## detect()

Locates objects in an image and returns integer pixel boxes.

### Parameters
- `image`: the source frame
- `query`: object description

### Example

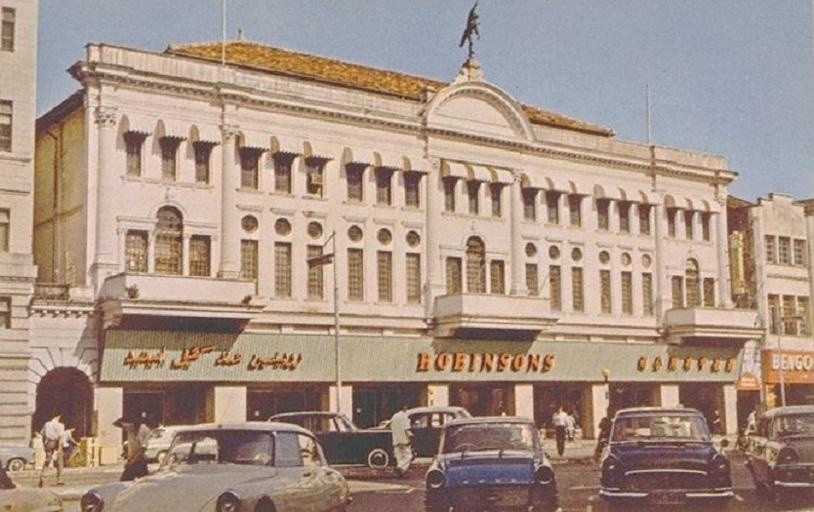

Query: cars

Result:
[120,424,219,463]
[600,407,736,504]
[80,420,352,512]
[0,445,35,474]
[0,468,64,512]
[738,406,814,496]
[267,407,472,469]
[422,414,559,512]
